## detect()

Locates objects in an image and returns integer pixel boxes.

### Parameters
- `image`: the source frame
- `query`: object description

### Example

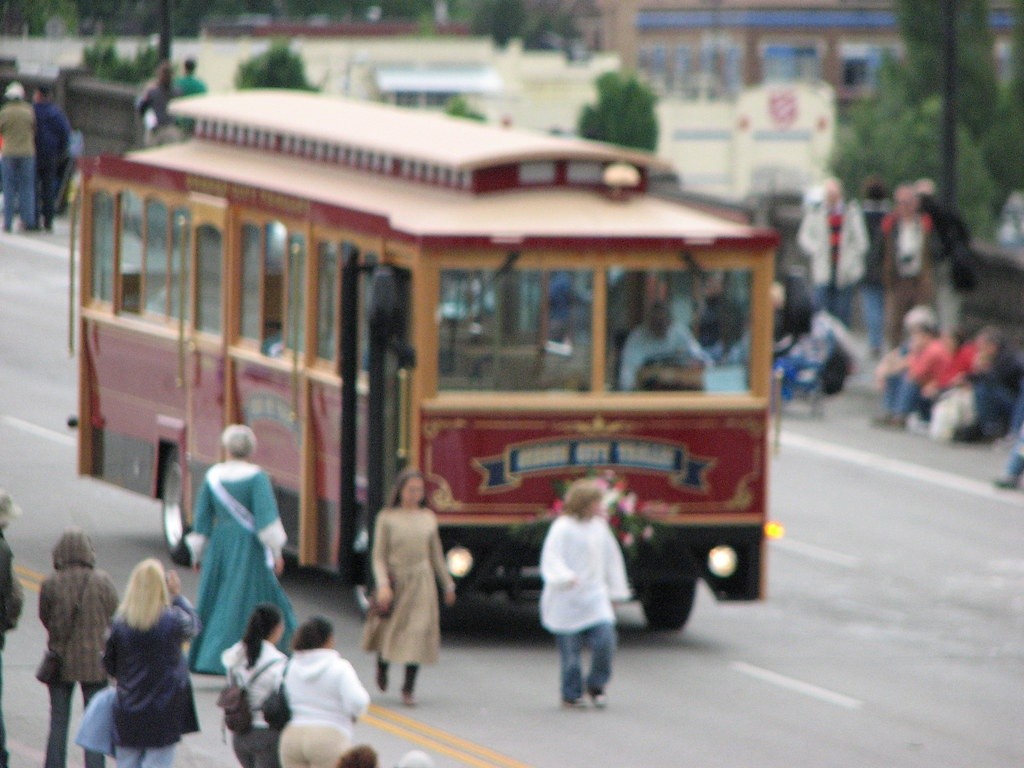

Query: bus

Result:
[67,85,782,634]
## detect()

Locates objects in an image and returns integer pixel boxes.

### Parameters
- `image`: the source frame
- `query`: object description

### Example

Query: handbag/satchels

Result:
[369,577,397,620]
[262,689,290,732]
[36,654,64,686]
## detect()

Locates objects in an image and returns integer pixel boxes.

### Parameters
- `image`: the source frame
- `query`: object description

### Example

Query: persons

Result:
[538,477,634,711]
[0,81,71,231]
[274,616,371,768]
[39,528,121,768]
[534,269,752,390]
[363,470,458,707]
[0,491,23,768]
[220,603,290,768]
[103,558,200,768]
[770,176,1024,488]
[337,744,435,768]
[182,423,299,676]
[139,59,207,147]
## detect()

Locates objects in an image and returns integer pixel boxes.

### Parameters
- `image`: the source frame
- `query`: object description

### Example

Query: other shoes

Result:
[592,694,608,708]
[562,697,587,709]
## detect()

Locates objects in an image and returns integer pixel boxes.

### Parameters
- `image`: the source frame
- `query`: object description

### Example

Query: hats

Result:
[5,83,25,101]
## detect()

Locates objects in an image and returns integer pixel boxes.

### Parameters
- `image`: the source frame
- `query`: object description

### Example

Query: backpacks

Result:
[216,684,253,733]
